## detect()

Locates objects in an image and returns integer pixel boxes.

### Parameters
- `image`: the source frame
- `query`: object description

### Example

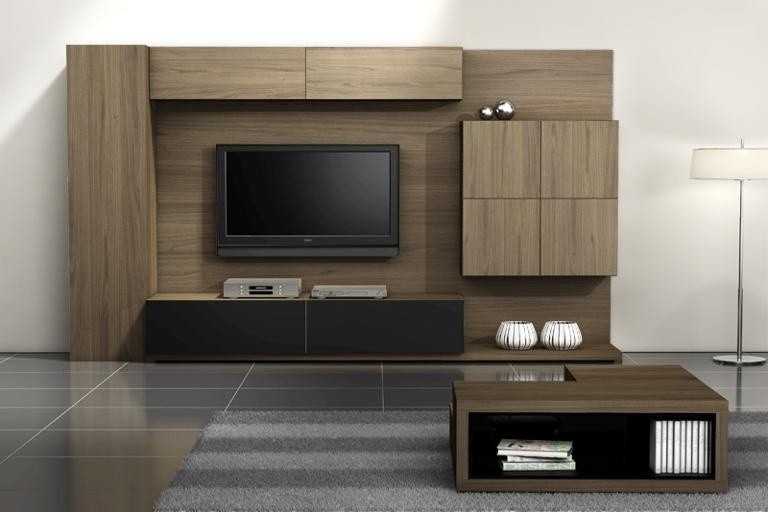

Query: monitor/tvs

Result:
[216,144,399,247]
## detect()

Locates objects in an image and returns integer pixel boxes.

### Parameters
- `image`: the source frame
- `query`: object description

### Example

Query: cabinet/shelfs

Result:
[149,47,462,99]
[145,294,463,355]
[460,120,619,276]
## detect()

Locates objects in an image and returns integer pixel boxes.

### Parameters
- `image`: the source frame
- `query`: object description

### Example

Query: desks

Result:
[450,365,729,494]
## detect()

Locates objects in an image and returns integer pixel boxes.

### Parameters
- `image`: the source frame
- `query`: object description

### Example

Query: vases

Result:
[496,316,538,349]
[541,320,583,350]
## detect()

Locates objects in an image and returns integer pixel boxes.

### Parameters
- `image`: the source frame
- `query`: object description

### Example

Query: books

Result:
[494,436,581,472]
[647,418,714,475]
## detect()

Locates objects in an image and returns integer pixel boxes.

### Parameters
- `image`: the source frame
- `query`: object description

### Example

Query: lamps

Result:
[689,140,768,365]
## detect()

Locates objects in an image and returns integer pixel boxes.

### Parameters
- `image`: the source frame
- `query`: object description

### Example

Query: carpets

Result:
[150,411,768,511]
